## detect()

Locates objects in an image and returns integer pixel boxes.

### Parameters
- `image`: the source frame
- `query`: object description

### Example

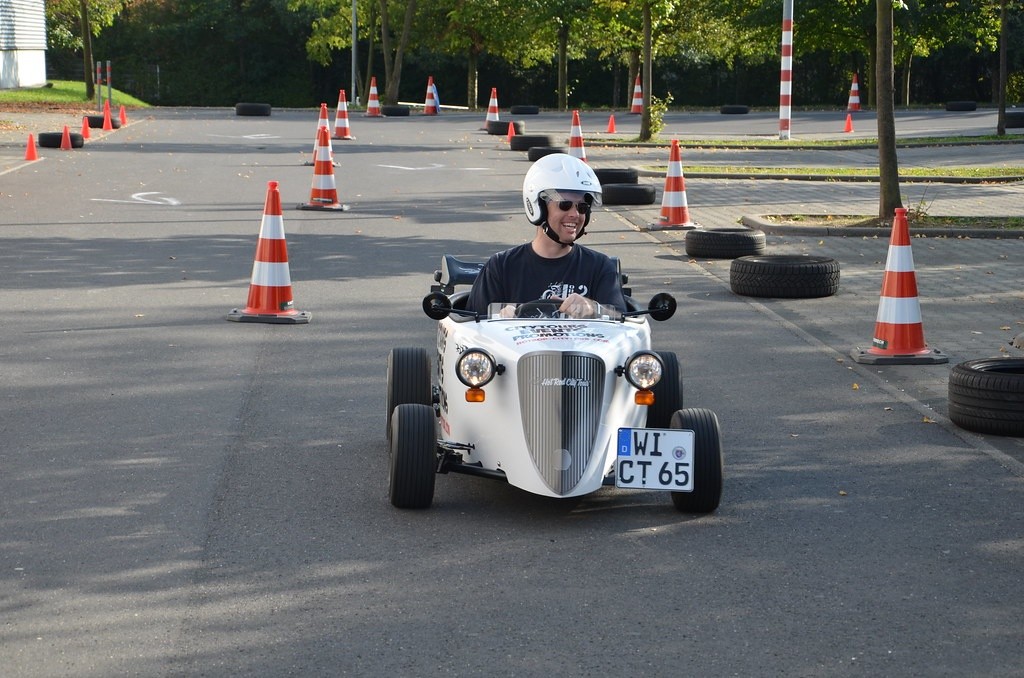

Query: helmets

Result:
[523,153,603,226]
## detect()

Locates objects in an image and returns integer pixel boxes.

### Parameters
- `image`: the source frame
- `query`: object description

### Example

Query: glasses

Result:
[553,199,590,214]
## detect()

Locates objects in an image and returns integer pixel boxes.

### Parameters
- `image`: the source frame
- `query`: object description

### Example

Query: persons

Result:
[466,152,628,322]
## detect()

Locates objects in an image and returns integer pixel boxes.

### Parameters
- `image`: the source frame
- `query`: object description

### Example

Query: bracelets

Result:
[594,301,601,320]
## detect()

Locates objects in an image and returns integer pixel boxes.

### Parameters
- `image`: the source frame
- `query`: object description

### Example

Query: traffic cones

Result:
[626,76,643,115]
[301,103,341,166]
[605,115,619,134]
[478,88,500,130]
[100,99,113,131]
[80,117,91,139]
[567,110,587,163]
[330,90,357,139]
[226,179,313,324]
[417,76,442,116]
[60,125,73,150]
[648,139,703,230]
[118,105,127,125]
[362,78,385,117]
[296,125,349,211]
[842,114,855,132]
[843,73,866,113]
[504,120,515,142]
[848,206,949,365]
[25,134,40,160]
